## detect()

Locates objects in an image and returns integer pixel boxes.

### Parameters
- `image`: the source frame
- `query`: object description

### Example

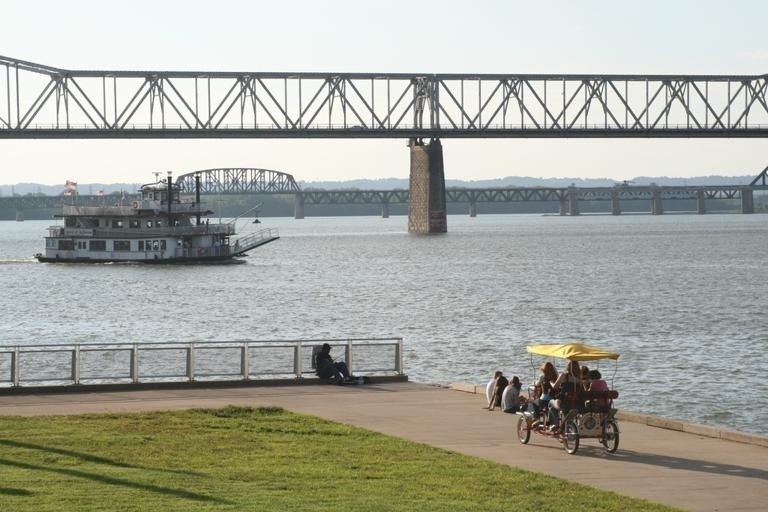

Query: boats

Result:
[34,167,283,264]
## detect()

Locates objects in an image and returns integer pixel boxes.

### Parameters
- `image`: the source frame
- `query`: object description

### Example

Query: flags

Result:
[63,180,77,198]
[96,190,104,197]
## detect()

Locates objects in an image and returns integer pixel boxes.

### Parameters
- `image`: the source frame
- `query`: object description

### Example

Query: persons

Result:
[482,357,612,434]
[313,343,355,386]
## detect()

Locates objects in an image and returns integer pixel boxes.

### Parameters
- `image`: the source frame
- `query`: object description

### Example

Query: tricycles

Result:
[513,343,621,456]
